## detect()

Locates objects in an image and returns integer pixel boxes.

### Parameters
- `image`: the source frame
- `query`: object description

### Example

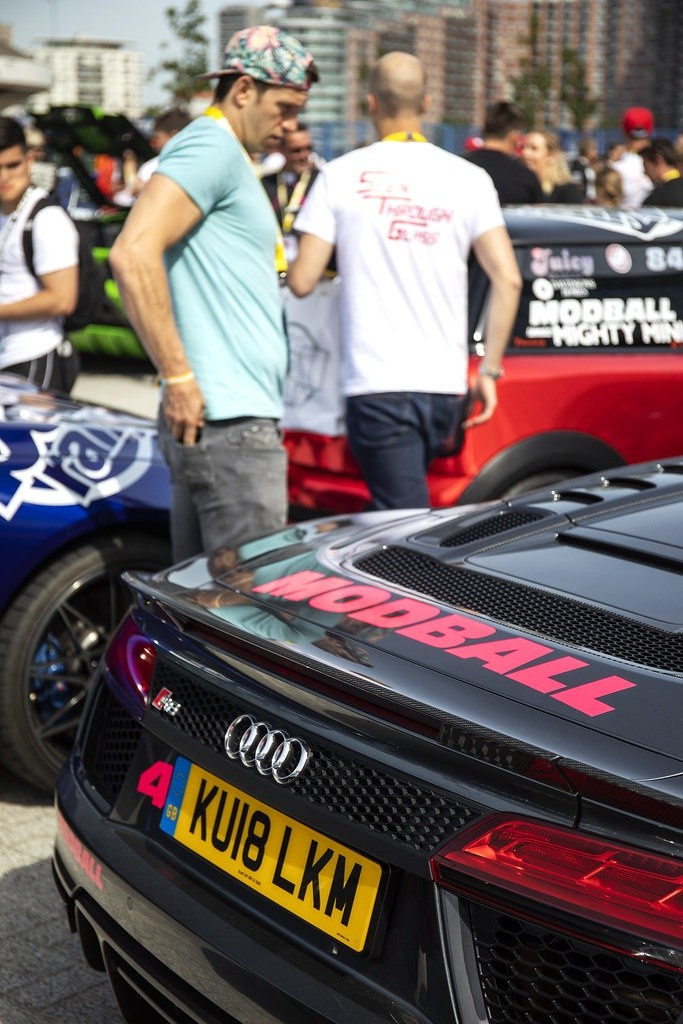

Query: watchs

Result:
[477,364,504,381]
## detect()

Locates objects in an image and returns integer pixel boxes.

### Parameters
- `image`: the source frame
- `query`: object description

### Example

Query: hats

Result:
[621,106,655,138]
[192,26,319,91]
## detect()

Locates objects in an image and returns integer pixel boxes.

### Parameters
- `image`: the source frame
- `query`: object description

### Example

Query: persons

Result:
[257,121,339,287]
[288,50,524,511]
[0,104,197,400]
[462,100,681,352]
[108,25,291,564]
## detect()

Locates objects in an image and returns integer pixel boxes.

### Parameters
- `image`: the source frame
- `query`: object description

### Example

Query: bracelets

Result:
[154,371,195,388]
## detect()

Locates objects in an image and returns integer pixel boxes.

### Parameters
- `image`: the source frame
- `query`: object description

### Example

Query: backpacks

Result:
[23,196,100,330]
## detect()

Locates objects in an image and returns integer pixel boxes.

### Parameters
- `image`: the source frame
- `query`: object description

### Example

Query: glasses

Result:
[289,145,314,153]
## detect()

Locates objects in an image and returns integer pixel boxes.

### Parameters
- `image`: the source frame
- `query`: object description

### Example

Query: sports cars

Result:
[52,455,682,1017]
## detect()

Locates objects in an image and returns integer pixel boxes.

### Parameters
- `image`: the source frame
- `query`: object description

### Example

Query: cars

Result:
[0,373,173,799]
[280,203,683,539]
